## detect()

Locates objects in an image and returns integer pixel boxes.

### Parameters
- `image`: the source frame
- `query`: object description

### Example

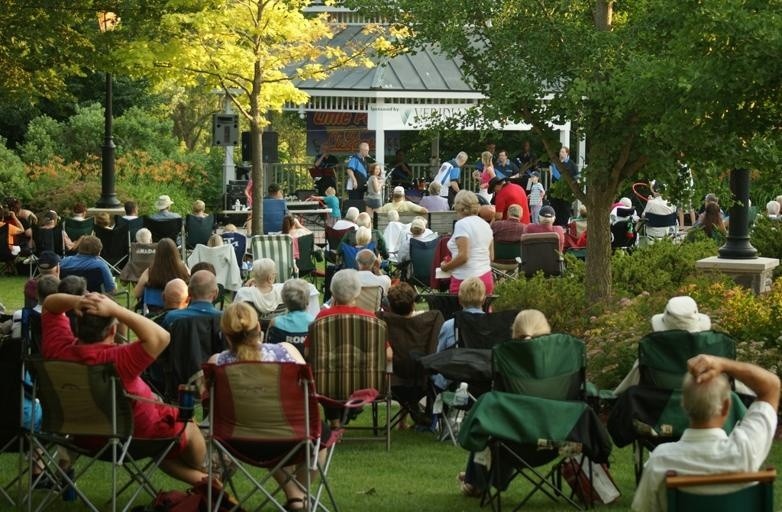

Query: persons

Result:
[512,308,551,340]
[551,145,579,184]
[422,277,487,430]
[366,163,384,209]
[514,139,541,169]
[314,143,339,169]
[434,152,590,295]
[766,196,782,218]
[2,174,438,510]
[494,149,519,176]
[607,178,729,248]
[344,142,370,200]
[474,142,497,172]
[612,296,712,395]
[631,354,782,512]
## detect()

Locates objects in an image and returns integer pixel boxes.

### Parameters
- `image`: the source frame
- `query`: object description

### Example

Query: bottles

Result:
[449,381,470,422]
[242,262,249,276]
[62,467,78,500]
[177,231,182,246]
[236,199,240,211]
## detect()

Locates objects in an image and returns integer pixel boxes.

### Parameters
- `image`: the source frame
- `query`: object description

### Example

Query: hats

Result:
[155,195,173,211]
[488,176,506,194]
[394,186,405,194]
[36,251,62,270]
[539,206,555,217]
[530,171,540,177]
[651,296,712,334]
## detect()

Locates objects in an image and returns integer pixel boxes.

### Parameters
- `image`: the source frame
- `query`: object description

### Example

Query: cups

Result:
[177,383,196,422]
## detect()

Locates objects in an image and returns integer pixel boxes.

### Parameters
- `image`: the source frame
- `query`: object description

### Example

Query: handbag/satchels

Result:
[552,452,622,506]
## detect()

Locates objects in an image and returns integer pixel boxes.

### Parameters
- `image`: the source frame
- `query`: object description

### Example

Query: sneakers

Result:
[458,471,466,481]
[461,480,474,497]
[211,449,241,487]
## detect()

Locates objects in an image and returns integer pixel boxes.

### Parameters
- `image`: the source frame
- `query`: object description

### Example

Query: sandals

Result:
[287,497,308,512]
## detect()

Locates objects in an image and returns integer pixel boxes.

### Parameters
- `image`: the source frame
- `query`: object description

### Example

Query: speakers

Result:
[242,130,277,164]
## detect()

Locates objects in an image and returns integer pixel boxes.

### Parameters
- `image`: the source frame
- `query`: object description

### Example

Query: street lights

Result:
[86,5,128,207]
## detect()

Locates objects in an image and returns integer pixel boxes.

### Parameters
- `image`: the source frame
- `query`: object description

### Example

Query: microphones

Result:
[367,154,376,161]
[516,158,522,164]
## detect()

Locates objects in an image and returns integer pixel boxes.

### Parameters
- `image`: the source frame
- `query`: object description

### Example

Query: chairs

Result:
[1,199,777,509]
